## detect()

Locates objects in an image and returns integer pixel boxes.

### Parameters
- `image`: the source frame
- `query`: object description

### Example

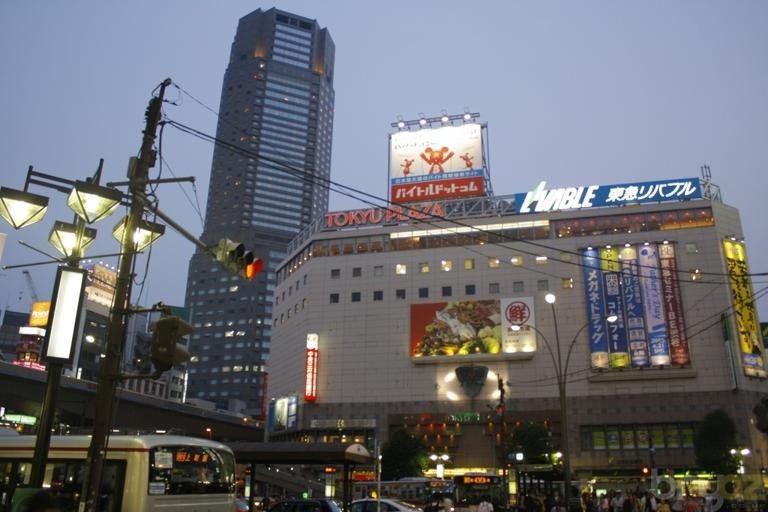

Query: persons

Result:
[429,486,714,512]
[260,496,270,512]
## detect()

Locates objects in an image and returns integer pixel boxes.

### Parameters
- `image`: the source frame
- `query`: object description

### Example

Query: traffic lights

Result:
[214,235,266,283]
[303,492,308,500]
[324,467,337,474]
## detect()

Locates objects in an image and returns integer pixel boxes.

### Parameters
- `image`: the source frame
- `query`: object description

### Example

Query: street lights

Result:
[0,157,166,512]
[510,292,617,512]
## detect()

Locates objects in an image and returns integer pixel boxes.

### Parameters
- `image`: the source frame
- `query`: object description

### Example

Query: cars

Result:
[266,497,343,512]
[344,495,423,512]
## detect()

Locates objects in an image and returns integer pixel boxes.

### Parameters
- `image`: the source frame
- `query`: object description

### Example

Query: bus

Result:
[451,471,501,512]
[1,423,239,508]
[347,475,458,505]
